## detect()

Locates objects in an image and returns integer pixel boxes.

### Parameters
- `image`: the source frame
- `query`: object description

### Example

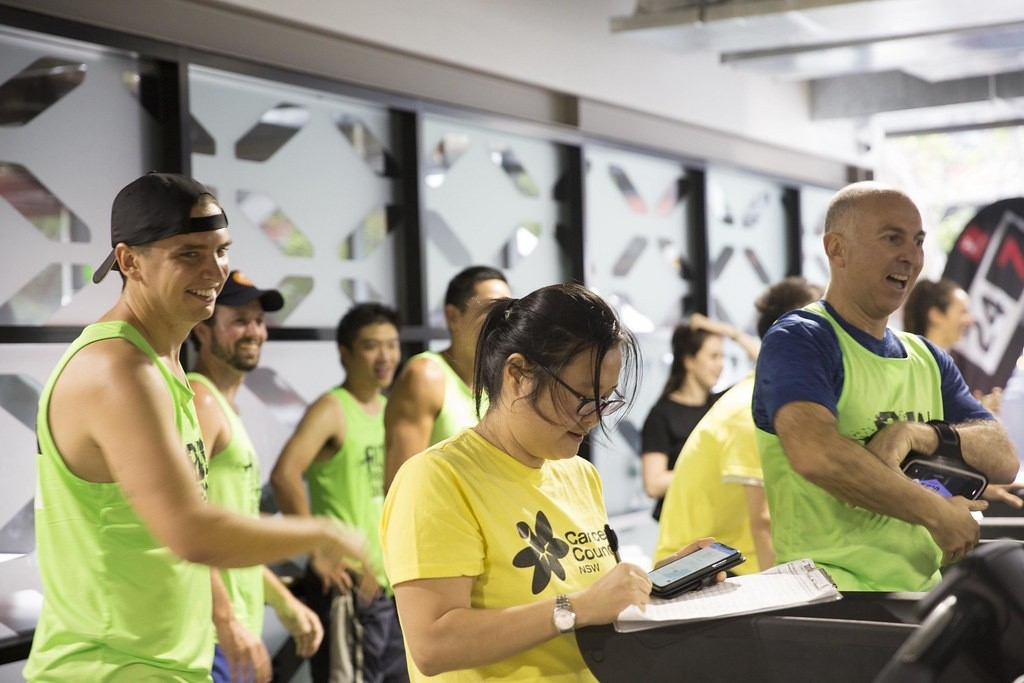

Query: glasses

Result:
[534,358,628,417]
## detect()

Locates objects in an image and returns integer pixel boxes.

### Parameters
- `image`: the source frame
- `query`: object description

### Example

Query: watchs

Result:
[927,421,958,458]
[552,594,575,634]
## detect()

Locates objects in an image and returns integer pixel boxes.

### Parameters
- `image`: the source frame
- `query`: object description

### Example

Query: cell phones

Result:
[647,541,741,593]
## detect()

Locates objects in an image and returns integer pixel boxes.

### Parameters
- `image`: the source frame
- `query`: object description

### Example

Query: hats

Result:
[92,170,230,283]
[215,271,286,311]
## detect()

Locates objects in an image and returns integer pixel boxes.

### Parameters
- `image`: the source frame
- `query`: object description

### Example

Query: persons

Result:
[384,266,514,498]
[379,283,726,683]
[755,280,826,338]
[904,279,972,350]
[654,374,776,579]
[184,268,323,683]
[20,168,368,683]
[750,181,1021,593]
[269,302,399,683]
[640,314,763,523]
